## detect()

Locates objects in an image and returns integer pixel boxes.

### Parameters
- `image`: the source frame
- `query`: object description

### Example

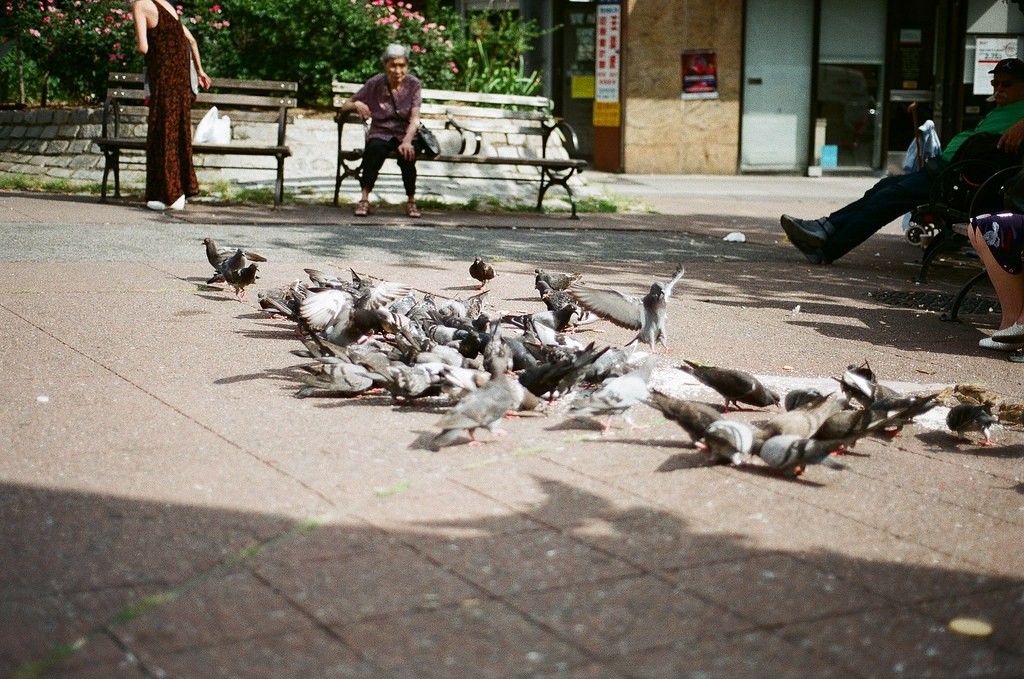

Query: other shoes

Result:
[147,194,185,210]
[978,321,1024,352]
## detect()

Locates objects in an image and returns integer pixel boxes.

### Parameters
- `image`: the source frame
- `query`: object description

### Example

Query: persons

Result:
[341,42,425,219]
[966,204,1024,353]
[778,58,1024,266]
[132,0,213,212]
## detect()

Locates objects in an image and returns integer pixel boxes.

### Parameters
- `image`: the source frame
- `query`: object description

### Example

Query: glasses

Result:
[991,80,1022,88]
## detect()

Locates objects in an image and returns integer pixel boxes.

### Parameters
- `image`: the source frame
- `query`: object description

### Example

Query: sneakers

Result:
[781,214,829,265]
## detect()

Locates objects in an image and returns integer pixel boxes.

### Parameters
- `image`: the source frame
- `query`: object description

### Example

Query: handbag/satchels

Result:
[194,106,231,147]
[190,53,198,99]
[415,125,441,156]
[143,60,198,106]
[999,169,1024,214]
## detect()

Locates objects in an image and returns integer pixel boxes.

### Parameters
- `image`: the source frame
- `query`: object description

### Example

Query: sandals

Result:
[355,200,370,216]
[407,201,421,218]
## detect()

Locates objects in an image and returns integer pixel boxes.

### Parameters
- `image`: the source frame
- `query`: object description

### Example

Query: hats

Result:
[988,58,1024,80]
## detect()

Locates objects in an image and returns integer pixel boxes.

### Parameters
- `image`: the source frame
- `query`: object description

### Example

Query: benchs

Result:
[332,82,588,221]
[919,158,1024,322]
[89,71,298,212]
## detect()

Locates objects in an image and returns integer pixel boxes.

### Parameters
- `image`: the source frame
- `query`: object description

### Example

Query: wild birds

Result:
[199,235,998,478]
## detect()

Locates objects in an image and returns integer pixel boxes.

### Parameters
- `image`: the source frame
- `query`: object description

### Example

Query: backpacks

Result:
[941,132,1024,211]
